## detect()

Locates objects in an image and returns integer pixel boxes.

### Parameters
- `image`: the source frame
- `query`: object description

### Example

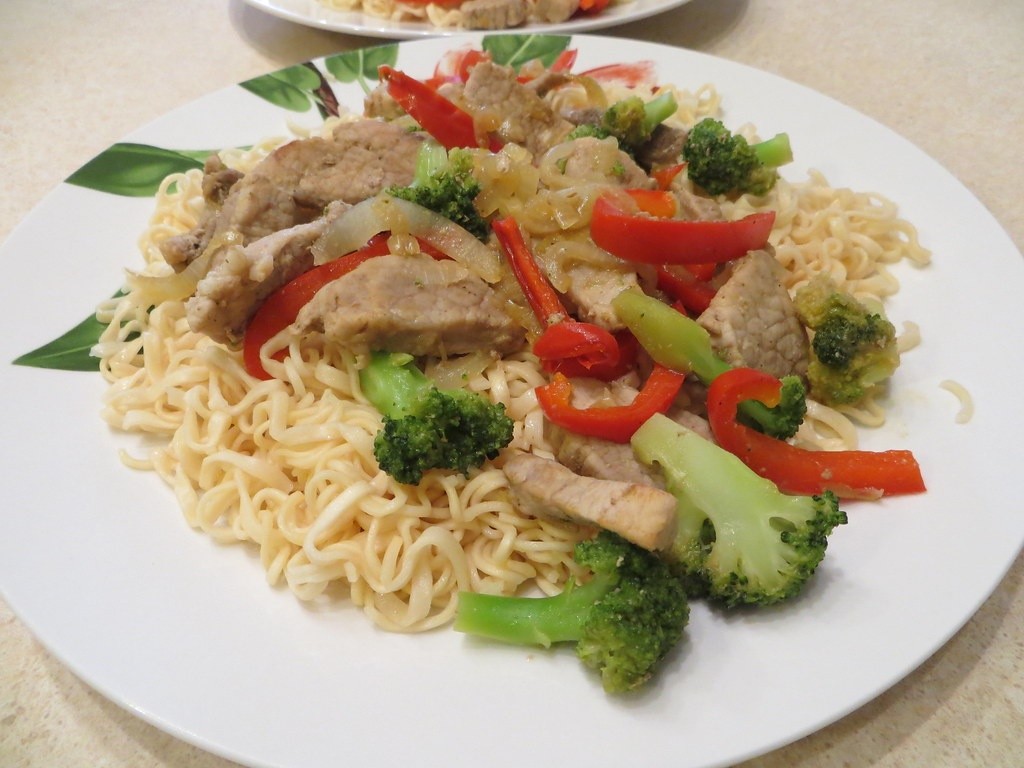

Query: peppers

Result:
[236,53,927,499]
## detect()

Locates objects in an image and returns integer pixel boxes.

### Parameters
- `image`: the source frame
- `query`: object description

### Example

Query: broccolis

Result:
[356,91,904,697]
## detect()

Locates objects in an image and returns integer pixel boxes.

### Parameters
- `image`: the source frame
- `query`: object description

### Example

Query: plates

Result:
[1,35,1024,768]
[243,0,687,39]
